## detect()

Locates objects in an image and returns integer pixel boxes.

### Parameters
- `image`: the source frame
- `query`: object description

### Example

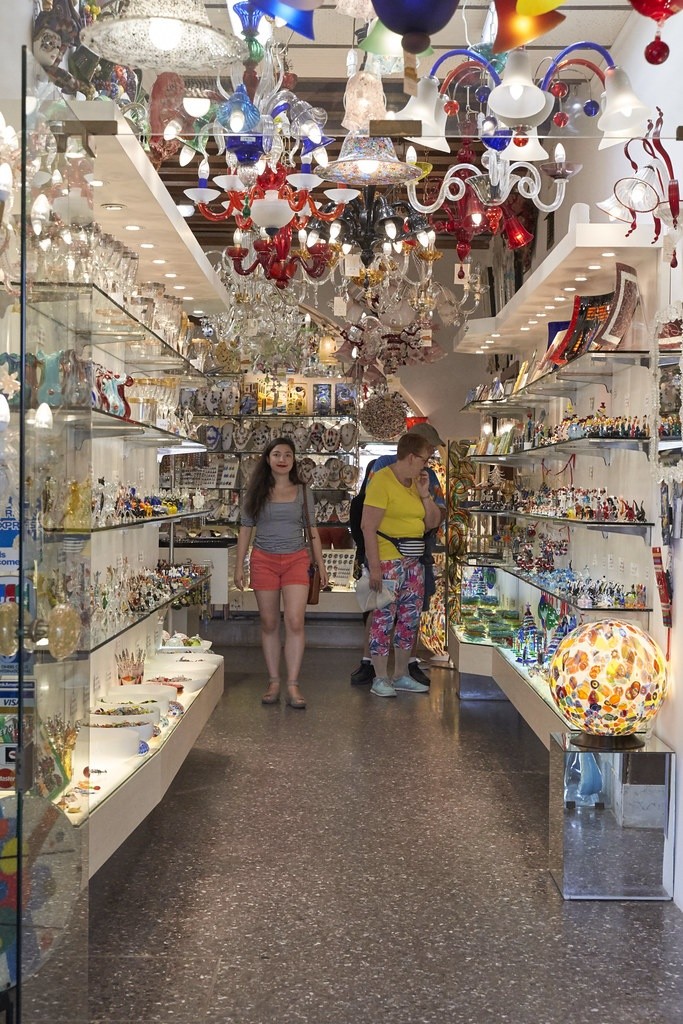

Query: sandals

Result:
[286,680,306,708]
[262,677,281,704]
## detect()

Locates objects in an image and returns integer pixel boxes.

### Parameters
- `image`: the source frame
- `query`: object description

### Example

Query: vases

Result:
[2,353,135,418]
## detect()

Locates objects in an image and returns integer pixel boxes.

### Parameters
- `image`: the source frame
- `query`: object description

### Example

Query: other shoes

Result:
[350,660,376,684]
[370,676,397,696]
[391,674,429,692]
[408,661,431,686]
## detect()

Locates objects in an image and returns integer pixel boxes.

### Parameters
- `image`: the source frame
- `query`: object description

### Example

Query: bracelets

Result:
[420,492,432,500]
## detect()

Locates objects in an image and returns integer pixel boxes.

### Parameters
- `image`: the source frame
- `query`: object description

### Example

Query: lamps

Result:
[0,0,683,370]
[550,617,671,749]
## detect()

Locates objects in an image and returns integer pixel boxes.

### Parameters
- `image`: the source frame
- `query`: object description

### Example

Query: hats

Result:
[409,423,446,448]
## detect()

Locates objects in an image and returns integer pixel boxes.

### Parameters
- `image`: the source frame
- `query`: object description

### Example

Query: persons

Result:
[350,434,436,697]
[318,456,351,551]
[349,422,446,686]
[233,438,329,708]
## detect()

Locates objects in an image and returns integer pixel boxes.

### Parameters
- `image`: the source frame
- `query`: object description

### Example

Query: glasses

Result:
[413,454,428,465]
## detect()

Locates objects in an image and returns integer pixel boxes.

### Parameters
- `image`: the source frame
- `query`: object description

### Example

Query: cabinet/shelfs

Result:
[1,277,223,992]
[158,376,363,619]
[447,201,675,747]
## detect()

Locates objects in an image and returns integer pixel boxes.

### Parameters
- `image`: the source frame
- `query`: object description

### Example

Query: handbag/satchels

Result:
[306,563,321,605]
[378,531,426,556]
